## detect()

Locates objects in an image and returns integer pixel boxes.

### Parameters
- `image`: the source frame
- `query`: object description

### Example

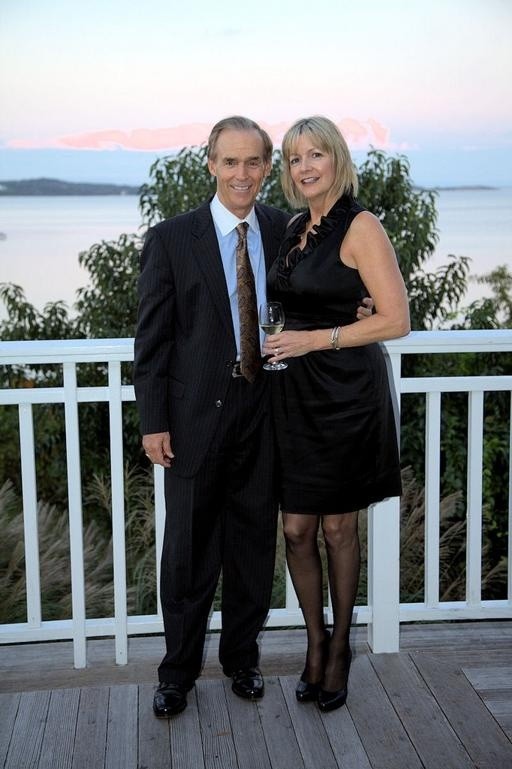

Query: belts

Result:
[231,353,273,378]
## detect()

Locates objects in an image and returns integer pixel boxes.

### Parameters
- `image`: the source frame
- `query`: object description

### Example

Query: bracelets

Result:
[328,326,343,351]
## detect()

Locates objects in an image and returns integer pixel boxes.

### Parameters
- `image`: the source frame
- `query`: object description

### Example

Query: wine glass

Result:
[257,302,289,372]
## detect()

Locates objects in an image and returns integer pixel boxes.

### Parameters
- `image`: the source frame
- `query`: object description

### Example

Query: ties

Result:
[235,222,264,388]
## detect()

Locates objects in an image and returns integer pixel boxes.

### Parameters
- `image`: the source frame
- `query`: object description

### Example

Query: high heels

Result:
[318,646,352,711]
[295,628,332,701]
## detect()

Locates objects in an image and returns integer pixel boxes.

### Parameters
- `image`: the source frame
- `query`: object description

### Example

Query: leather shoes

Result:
[152,679,194,718]
[221,666,266,701]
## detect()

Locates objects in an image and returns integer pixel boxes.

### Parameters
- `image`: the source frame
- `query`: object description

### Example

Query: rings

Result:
[273,347,279,354]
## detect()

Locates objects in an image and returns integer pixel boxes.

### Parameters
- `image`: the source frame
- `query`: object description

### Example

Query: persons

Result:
[261,116,411,711]
[135,116,373,721]
